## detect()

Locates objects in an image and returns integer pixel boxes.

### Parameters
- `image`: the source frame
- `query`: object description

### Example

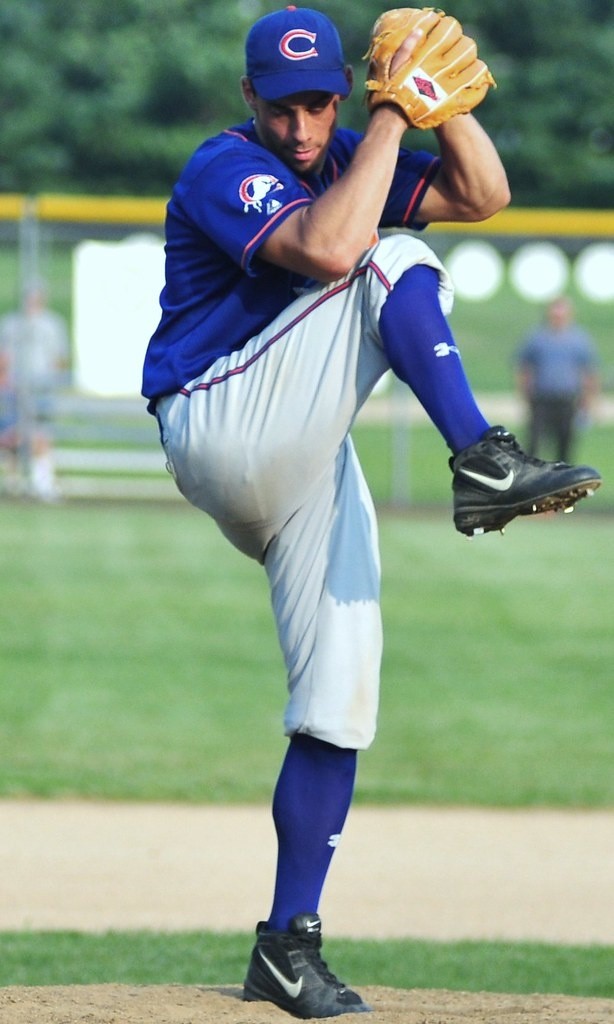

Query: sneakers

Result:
[242,913,370,1018]
[448,426,601,541]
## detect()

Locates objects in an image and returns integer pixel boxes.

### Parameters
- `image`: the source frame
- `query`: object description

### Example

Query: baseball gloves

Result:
[363,5,494,133]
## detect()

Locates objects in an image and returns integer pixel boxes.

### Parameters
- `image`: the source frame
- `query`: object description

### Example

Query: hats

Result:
[245,6,350,101]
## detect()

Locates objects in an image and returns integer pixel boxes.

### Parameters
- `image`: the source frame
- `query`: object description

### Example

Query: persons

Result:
[0,272,70,502]
[514,295,600,519]
[141,5,599,1019]
